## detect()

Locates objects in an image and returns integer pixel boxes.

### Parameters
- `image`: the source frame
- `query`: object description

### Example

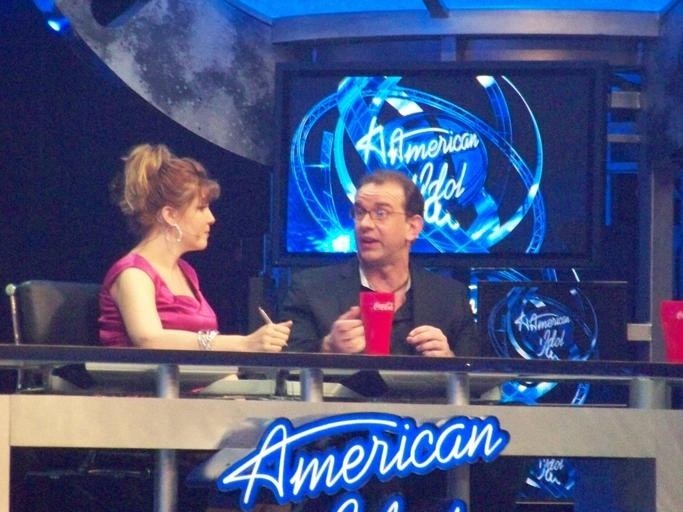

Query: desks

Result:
[0,337,683,512]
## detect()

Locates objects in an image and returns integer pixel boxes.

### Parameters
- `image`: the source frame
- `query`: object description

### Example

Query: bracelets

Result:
[198,329,221,352]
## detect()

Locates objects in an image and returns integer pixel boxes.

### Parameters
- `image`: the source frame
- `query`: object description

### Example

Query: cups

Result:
[660,300,683,364]
[359,291,396,357]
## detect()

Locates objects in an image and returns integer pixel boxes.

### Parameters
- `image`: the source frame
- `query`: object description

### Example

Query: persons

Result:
[94,140,297,512]
[277,168,539,512]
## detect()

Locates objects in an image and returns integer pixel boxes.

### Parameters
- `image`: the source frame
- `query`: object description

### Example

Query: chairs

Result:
[6,280,166,509]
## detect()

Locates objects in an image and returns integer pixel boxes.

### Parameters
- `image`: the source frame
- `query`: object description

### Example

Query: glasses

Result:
[350,205,405,220]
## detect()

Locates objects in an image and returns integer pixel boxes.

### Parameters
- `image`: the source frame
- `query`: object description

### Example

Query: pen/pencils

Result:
[258,306,288,347]
